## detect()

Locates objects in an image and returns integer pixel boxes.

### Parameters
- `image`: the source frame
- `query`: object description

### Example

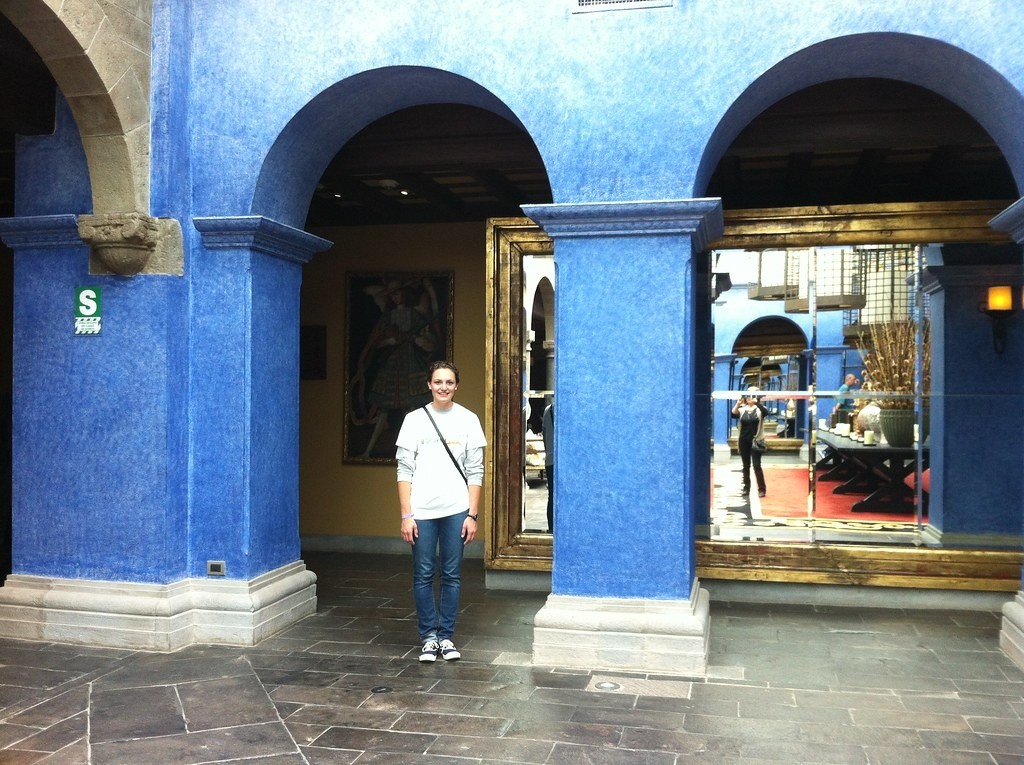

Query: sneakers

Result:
[419,641,439,662]
[439,639,461,660]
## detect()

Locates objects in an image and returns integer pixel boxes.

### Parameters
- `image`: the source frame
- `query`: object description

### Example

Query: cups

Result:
[864,431,874,444]
[818,419,826,427]
[842,424,850,435]
[836,423,844,433]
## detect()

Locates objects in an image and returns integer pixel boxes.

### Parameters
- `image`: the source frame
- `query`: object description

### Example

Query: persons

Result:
[394,360,488,662]
[542,403,553,532]
[730,386,768,497]
[833,373,855,413]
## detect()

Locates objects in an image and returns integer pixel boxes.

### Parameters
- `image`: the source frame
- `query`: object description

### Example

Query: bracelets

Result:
[402,513,414,518]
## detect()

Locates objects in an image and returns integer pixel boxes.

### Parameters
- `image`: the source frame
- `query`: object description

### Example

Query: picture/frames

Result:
[341,270,457,466]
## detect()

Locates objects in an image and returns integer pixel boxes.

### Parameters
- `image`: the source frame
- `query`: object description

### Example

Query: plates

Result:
[862,443,877,447]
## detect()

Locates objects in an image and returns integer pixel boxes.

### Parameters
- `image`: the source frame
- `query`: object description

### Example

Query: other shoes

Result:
[743,486,750,496]
[758,489,765,497]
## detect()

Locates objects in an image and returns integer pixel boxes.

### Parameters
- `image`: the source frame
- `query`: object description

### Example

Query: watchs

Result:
[467,513,480,521]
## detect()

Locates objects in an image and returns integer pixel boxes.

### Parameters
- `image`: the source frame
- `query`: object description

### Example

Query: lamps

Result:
[978,278,1020,355]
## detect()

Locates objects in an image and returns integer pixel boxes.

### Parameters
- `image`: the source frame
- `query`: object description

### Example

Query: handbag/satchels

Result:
[752,436,767,454]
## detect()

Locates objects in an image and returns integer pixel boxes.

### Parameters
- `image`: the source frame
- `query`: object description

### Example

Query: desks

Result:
[801,429,930,513]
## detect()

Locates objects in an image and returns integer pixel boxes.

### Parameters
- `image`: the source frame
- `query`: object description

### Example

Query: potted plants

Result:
[856,313,932,447]
